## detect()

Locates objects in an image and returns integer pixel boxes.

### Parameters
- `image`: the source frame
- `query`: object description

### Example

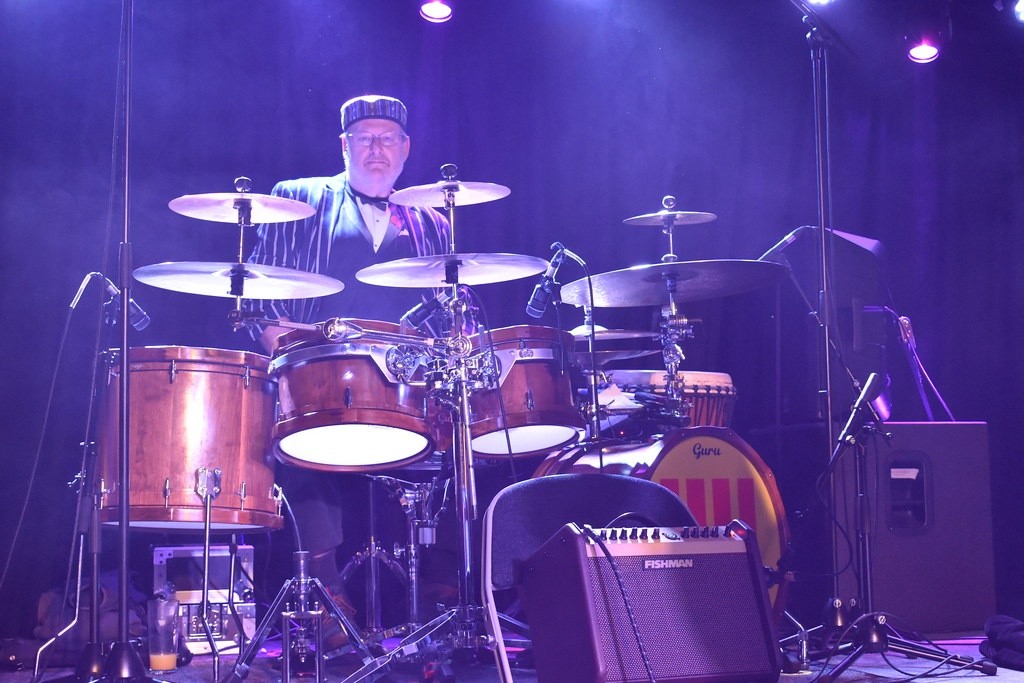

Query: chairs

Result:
[482,473,697,682]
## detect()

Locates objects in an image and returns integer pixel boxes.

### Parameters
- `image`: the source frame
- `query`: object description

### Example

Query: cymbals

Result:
[560,260,792,308]
[570,324,664,365]
[132,261,344,301]
[167,194,315,223]
[389,181,512,208]
[622,212,717,225]
[355,252,551,287]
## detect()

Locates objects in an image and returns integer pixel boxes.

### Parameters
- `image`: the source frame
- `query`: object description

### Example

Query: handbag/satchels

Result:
[34,569,147,641]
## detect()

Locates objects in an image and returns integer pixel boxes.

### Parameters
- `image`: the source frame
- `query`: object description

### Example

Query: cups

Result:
[147,599,180,674]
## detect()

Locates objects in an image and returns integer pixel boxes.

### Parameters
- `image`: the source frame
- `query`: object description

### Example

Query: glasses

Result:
[347,131,403,146]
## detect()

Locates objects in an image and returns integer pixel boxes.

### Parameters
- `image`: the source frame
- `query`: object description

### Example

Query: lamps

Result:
[419,0,458,25]
[900,14,953,62]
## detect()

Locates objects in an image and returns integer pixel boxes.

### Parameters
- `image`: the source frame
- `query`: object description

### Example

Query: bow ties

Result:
[361,196,386,211]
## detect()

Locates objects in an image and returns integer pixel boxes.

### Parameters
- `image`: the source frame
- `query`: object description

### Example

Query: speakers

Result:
[735,226,995,637]
[515,518,781,683]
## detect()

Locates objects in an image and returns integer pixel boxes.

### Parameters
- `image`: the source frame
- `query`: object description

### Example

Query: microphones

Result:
[97,272,150,331]
[406,284,461,330]
[756,227,805,263]
[526,251,563,319]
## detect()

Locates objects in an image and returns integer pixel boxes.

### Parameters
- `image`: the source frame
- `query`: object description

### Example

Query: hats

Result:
[340,95,407,131]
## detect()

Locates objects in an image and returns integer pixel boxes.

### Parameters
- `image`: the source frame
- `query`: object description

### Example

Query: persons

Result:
[241,96,454,645]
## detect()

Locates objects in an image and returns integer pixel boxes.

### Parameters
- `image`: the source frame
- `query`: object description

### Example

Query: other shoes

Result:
[315,586,358,649]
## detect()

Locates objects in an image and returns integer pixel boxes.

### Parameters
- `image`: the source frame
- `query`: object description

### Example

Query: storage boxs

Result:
[173,603,256,655]
[143,544,254,604]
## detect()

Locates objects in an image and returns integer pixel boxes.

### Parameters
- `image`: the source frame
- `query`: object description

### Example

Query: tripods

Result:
[767,251,998,683]
[223,191,531,683]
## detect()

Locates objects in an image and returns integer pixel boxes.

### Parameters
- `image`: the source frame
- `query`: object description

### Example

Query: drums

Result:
[531,427,792,626]
[467,326,587,459]
[598,369,735,426]
[271,319,436,471]
[97,346,283,534]
